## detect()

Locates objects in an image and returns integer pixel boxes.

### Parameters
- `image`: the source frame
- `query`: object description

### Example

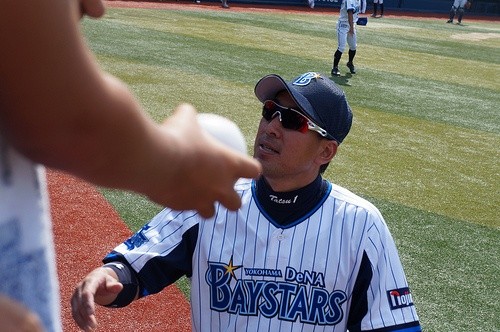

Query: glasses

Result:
[261,100,342,147]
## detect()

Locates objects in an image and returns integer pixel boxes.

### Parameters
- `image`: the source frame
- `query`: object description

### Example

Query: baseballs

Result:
[196,113,246,157]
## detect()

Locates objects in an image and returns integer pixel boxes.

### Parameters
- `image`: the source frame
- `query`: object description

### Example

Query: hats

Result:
[254,70,353,145]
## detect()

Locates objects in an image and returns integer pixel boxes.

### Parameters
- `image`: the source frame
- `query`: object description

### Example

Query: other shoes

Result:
[346,62,356,73]
[332,68,341,75]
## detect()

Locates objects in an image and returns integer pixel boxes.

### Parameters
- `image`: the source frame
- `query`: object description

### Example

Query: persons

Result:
[447,0,468,25]
[359,0,367,14]
[0,0,262,332]
[371,0,384,19]
[71,71,423,332]
[331,0,367,77]
